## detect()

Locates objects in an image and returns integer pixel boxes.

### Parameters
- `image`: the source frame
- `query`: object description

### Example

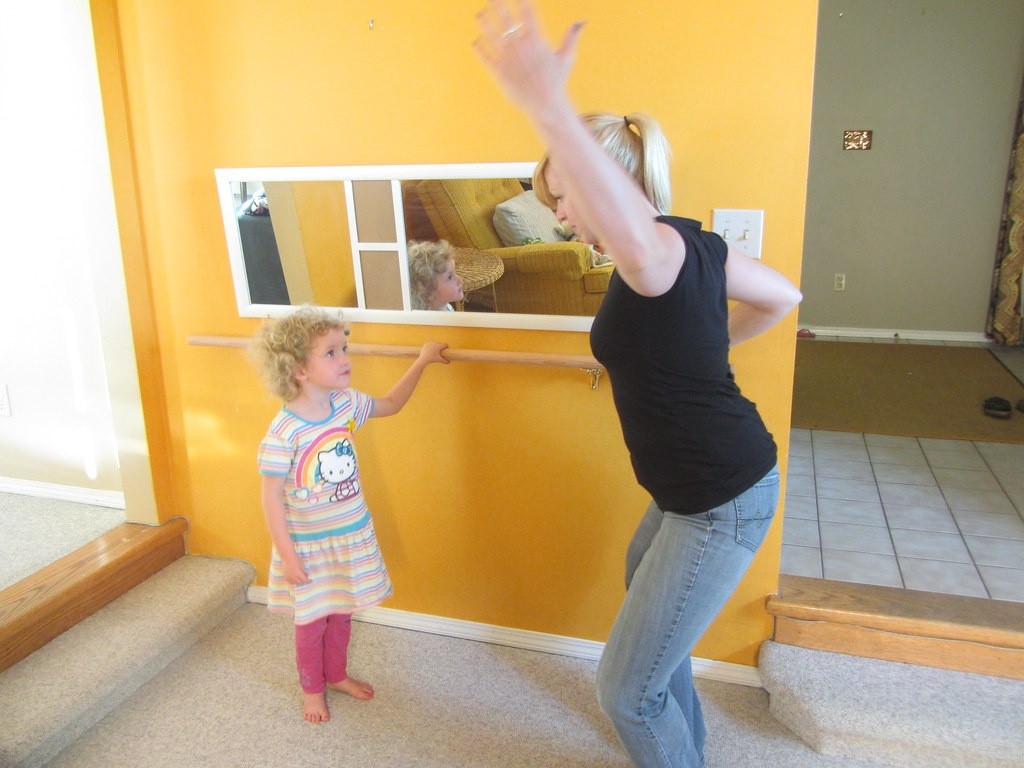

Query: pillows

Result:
[492,190,566,247]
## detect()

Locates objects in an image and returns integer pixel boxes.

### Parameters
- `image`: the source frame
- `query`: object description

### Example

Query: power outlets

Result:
[835,273,845,290]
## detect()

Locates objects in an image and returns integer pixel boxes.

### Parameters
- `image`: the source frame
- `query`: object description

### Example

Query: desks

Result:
[453,244,505,312]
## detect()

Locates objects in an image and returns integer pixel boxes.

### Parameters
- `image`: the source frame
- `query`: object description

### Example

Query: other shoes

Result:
[984,396,1012,417]
[1016,399,1024,412]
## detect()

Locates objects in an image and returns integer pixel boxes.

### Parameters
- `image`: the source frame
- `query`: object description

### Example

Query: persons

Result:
[256,303,450,723]
[476,0,804,768]
[409,240,465,311]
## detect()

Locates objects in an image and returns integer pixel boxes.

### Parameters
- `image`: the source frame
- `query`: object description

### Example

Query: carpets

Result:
[791,339,1024,445]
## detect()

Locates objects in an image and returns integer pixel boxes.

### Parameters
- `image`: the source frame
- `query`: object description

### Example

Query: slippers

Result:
[797,328,815,337]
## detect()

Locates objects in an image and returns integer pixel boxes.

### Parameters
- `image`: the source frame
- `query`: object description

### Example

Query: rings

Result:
[501,23,524,38]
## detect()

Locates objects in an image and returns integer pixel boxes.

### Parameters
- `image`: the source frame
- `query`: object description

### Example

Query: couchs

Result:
[415,179,617,317]
